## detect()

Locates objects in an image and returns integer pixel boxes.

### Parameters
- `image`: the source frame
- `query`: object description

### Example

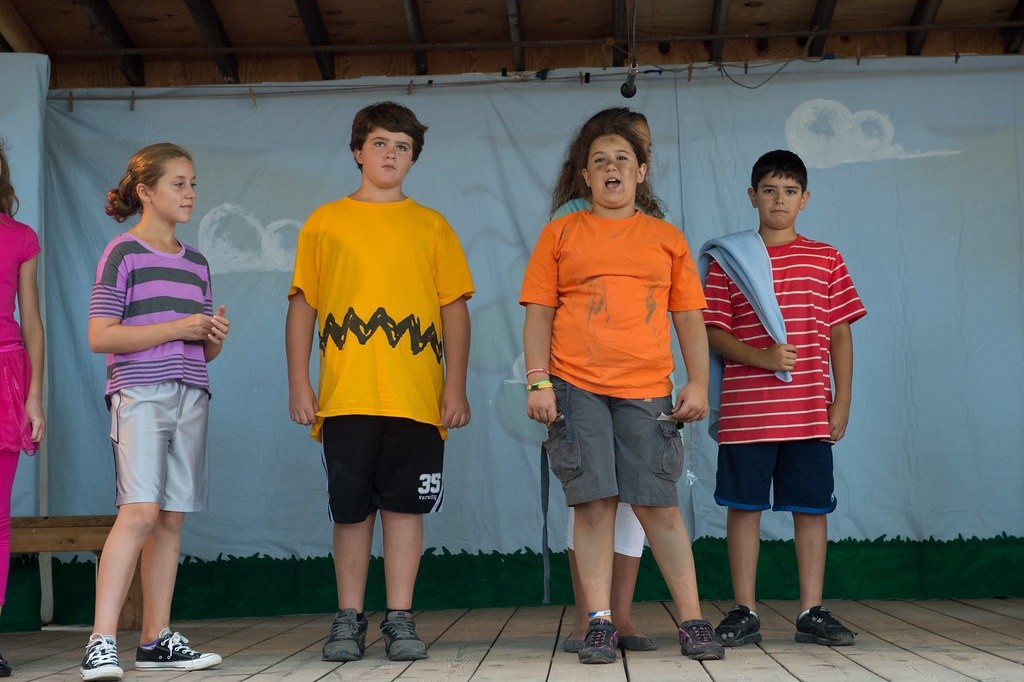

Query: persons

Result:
[77,141,231,682]
[519,123,725,665]
[701,149,868,646]
[286,101,476,662]
[0,138,48,678]
[551,107,687,655]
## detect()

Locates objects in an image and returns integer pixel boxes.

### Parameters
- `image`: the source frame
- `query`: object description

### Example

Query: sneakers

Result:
[714,604,763,646]
[135,631,222,671]
[379,611,430,659]
[678,619,725,661]
[578,618,618,663]
[80,633,124,681]
[323,609,367,660]
[794,605,855,645]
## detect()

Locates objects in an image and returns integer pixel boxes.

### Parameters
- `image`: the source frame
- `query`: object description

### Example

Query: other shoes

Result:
[617,632,659,652]
[563,630,584,653]
[0,654,12,677]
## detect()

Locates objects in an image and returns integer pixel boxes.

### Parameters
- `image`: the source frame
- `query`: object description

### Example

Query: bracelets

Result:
[526,379,555,392]
[523,368,549,379]
